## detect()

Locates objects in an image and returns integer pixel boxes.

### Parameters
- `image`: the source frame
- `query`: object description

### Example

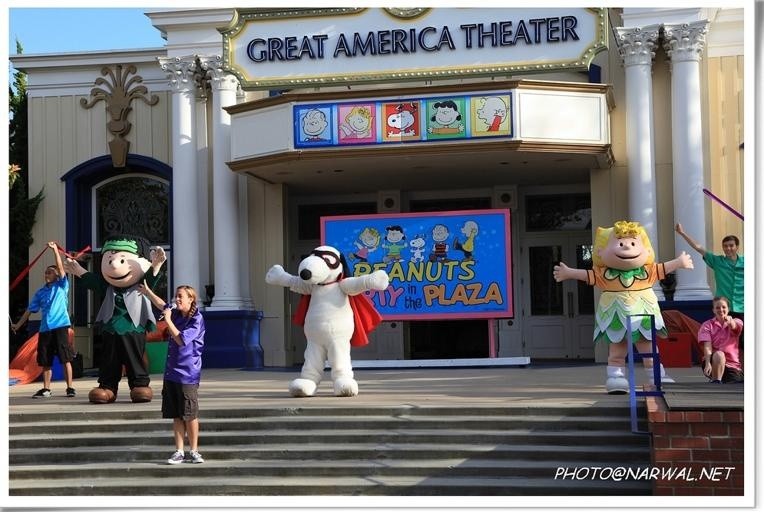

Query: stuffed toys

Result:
[62,233,166,404]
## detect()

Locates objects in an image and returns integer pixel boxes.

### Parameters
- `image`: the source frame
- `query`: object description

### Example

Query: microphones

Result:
[157,303,177,322]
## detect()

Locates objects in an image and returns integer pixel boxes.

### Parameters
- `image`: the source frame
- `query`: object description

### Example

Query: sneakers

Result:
[33,389,51,398]
[168,451,184,464]
[189,450,203,462]
[67,387,75,396]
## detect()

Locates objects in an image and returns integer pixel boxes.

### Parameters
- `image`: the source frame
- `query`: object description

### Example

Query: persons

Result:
[674,222,744,317]
[697,296,744,385]
[10,240,77,398]
[137,278,205,464]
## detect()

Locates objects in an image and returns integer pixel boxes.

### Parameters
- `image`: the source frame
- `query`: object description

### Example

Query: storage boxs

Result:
[656,330,693,369]
